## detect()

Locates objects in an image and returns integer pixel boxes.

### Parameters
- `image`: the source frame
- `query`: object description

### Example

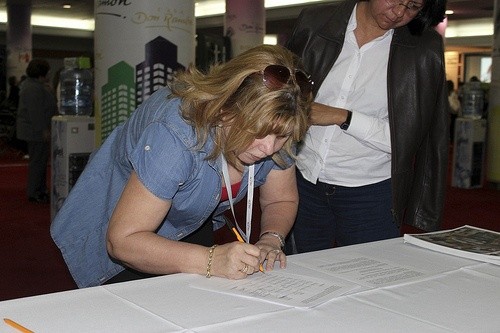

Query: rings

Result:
[240,263,246,272]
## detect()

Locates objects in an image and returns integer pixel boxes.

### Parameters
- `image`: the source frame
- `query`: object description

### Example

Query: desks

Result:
[0,238,500,333]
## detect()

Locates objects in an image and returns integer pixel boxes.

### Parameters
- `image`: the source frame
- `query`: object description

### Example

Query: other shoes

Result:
[28,191,51,203]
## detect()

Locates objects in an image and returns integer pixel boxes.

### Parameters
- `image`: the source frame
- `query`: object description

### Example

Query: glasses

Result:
[248,65,316,91]
[384,0,423,18]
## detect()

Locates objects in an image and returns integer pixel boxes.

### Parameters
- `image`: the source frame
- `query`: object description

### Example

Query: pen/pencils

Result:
[2,318,33,333]
[231,227,264,273]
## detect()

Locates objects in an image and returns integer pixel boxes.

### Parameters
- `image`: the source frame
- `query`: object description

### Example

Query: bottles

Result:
[59,69,93,116]
[461,82,483,119]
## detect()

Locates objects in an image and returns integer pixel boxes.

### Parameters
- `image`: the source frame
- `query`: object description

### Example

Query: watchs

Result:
[259,231,286,247]
[341,109,353,130]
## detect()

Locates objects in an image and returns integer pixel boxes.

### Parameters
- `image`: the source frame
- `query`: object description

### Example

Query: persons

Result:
[16,58,56,203]
[279,1,449,250]
[7,75,21,151]
[50,44,316,292]
[447,76,490,150]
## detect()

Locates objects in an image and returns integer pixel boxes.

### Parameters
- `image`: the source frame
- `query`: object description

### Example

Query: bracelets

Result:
[206,244,217,278]
